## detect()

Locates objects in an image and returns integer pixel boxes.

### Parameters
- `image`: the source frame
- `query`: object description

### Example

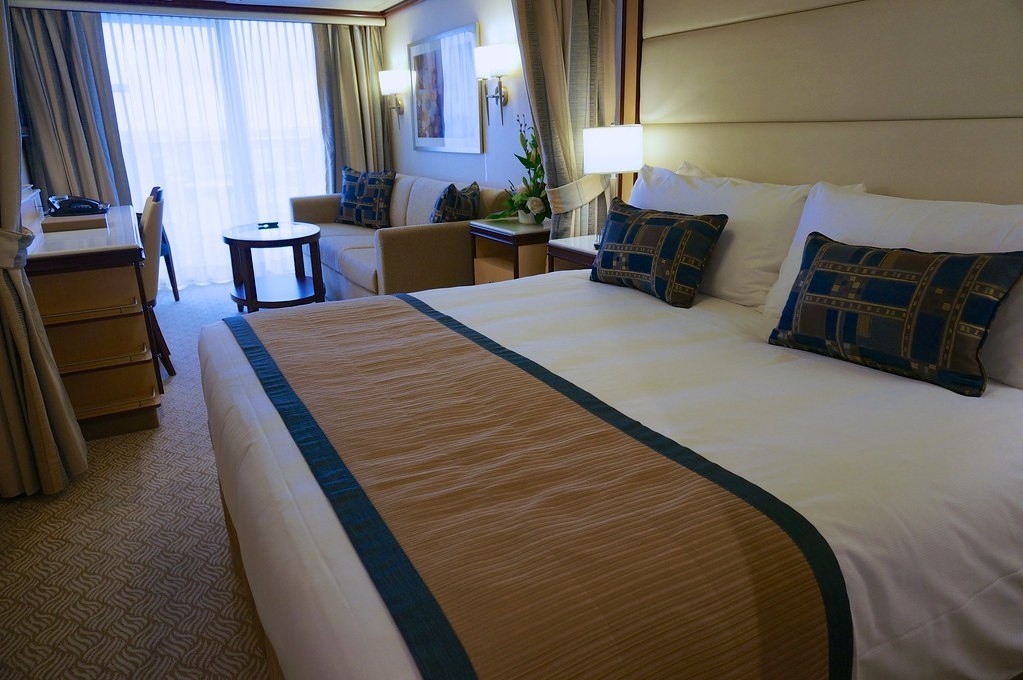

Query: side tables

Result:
[223,219,326,315]
[470,219,551,285]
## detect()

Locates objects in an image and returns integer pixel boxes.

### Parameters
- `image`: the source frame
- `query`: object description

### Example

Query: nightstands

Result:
[545,233,603,273]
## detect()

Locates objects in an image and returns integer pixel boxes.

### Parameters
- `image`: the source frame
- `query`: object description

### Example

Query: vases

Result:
[516,209,537,225]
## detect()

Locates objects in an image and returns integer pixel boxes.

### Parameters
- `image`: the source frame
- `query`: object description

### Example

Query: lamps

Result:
[379,70,405,130]
[583,122,644,249]
[474,44,510,126]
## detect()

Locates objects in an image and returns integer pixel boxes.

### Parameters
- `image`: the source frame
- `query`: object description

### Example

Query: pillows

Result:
[630,164,814,313]
[336,165,397,230]
[590,197,730,309]
[762,179,1023,391]
[766,231,1023,397]
[428,181,481,224]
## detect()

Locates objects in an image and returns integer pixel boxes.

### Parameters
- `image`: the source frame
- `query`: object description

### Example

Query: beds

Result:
[200,272,1023,680]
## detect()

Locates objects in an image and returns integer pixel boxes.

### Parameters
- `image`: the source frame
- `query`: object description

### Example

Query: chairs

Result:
[138,185,176,377]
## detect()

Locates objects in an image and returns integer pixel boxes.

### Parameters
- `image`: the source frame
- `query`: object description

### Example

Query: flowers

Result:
[486,114,552,225]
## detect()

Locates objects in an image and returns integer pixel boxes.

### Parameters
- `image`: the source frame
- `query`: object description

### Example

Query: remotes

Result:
[258,222,278,226]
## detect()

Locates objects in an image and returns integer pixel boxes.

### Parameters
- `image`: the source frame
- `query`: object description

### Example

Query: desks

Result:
[24,205,162,442]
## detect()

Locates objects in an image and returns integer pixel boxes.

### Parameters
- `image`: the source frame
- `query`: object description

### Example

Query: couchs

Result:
[290,173,514,295]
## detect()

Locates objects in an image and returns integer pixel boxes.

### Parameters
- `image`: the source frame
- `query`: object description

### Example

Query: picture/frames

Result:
[406,21,483,155]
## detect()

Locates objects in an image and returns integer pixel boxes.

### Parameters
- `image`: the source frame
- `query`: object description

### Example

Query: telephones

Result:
[43,194,109,217]
